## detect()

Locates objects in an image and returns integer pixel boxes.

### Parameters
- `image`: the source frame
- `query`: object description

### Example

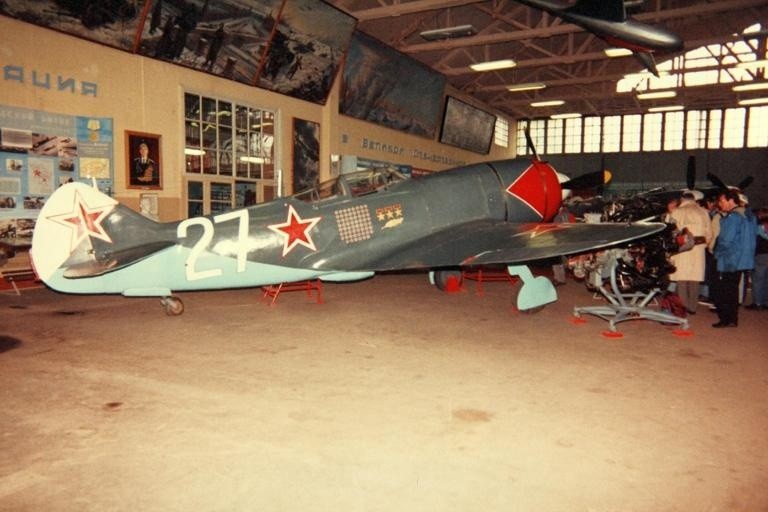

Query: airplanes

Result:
[32,126,667,315]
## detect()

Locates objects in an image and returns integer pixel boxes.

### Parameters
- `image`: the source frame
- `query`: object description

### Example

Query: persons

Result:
[662,190,768,328]
[263,49,304,81]
[131,138,156,185]
[148,0,226,72]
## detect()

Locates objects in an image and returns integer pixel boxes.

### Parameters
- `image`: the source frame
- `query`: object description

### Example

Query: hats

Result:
[682,189,705,201]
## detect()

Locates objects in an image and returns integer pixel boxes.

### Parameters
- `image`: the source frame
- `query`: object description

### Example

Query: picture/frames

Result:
[124,129,164,191]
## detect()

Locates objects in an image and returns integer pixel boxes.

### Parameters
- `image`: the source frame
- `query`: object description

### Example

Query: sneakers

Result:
[713,320,738,328]
